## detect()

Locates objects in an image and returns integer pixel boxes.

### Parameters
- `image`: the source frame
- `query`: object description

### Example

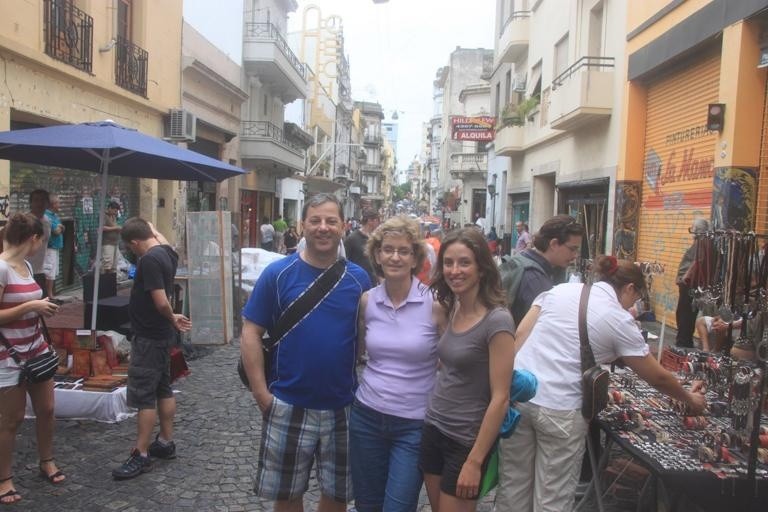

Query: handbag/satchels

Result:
[235,258,349,390]
[1,315,60,384]
[579,284,610,420]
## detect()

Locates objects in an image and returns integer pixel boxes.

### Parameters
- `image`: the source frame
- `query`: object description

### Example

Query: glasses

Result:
[565,244,579,252]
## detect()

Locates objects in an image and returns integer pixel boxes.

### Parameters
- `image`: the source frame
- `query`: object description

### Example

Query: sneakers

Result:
[112,449,152,478]
[148,434,176,459]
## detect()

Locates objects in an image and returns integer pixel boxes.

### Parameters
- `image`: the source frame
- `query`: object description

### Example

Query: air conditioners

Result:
[170,108,196,145]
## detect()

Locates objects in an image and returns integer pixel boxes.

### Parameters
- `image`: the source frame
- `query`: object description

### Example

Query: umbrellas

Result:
[0,118,252,351]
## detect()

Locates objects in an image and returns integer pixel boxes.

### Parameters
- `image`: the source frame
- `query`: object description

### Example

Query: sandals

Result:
[38,457,66,483]
[0,476,22,505]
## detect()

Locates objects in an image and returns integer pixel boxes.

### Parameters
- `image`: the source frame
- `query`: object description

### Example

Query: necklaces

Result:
[727,367,762,430]
[691,229,768,325]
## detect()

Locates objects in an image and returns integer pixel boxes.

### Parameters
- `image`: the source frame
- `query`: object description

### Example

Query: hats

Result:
[362,209,385,218]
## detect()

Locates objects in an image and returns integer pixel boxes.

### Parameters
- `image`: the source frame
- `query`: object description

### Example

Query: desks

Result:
[574,352,764,512]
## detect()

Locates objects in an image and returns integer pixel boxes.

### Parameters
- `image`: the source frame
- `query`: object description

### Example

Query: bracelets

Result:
[606,391,625,404]
[683,416,706,431]
[621,376,638,390]
[616,413,643,432]
[699,432,746,463]
[671,397,693,417]
[681,352,727,387]
[757,425,768,464]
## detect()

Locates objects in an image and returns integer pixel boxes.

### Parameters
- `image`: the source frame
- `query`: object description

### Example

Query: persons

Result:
[101,201,121,274]
[416,230,443,287]
[348,216,449,512]
[0,212,66,505]
[673,219,710,345]
[344,208,386,286]
[515,221,532,255]
[231,224,239,251]
[24,190,52,299]
[386,198,428,215]
[283,225,300,253]
[260,216,275,251]
[487,227,496,240]
[240,193,373,511]
[42,193,66,299]
[417,230,515,512]
[273,214,288,253]
[112,217,193,480]
[500,215,585,326]
[493,255,706,511]
